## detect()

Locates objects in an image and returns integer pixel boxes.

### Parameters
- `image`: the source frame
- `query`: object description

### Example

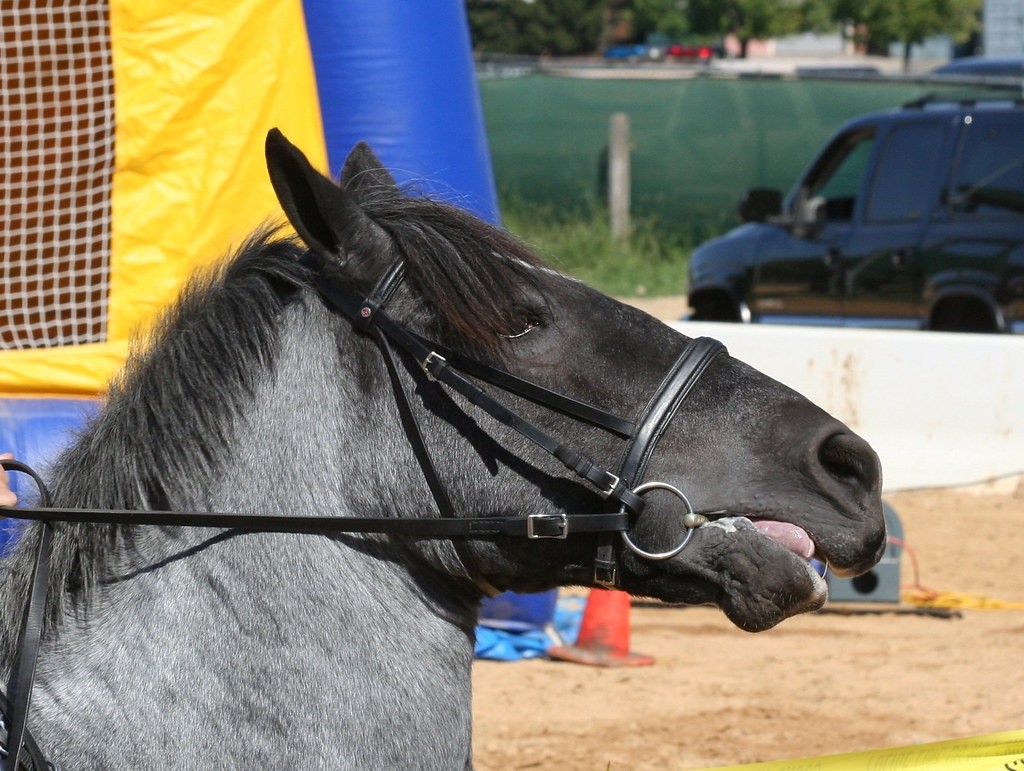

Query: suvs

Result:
[686,86,1024,335]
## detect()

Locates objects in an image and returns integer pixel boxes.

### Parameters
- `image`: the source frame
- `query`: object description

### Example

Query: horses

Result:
[0,129,888,771]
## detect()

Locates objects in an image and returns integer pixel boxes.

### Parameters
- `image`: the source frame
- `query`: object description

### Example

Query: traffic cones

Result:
[550,586,656,671]
